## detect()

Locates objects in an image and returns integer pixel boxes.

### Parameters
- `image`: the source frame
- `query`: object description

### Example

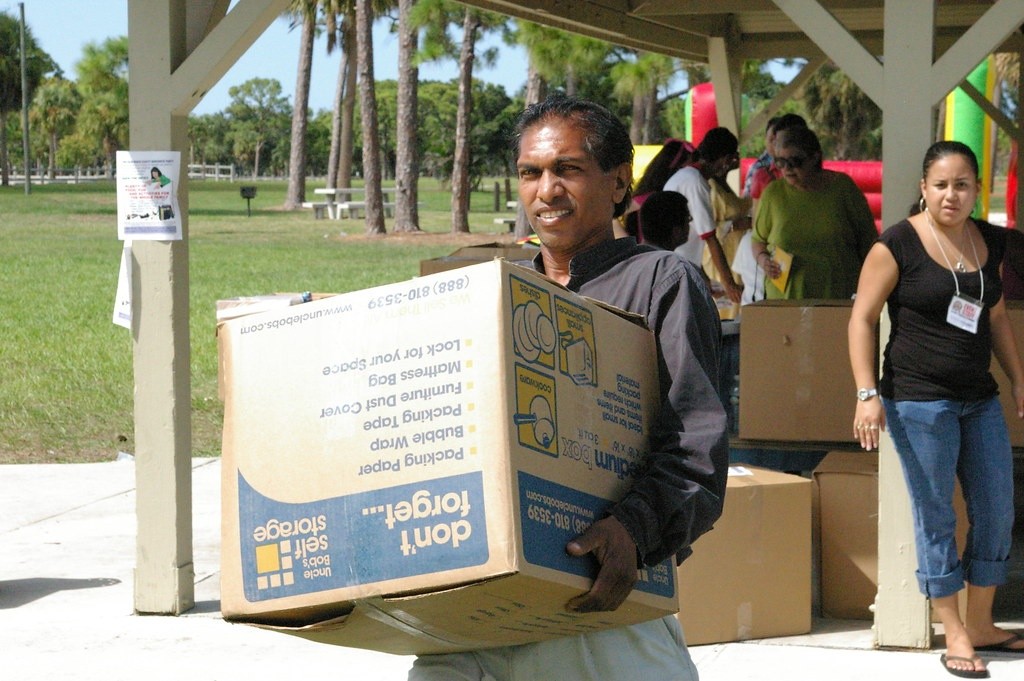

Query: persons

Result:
[664,127,743,302]
[849,138,1023,677]
[639,191,691,251]
[751,127,879,300]
[751,114,807,226]
[145,167,171,187]
[706,151,751,287]
[409,97,729,681]
[613,140,695,241]
[744,118,782,195]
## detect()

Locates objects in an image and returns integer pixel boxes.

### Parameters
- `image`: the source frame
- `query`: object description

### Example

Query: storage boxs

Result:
[217,242,683,655]
[983,295,1024,448]
[662,462,814,650]
[815,451,973,626]
[426,240,540,275]
[739,296,878,446]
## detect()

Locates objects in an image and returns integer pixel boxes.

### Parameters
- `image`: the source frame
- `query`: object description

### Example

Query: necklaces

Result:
[928,215,966,272]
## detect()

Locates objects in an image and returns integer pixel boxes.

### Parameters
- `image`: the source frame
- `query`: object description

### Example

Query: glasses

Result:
[774,156,815,170]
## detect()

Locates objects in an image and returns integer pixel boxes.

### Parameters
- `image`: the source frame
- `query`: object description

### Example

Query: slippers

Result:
[940,653,987,677]
[973,632,1024,652]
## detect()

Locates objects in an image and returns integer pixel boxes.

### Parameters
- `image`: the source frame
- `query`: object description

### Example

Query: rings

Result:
[870,426,879,429]
[864,426,870,429]
[857,423,863,429]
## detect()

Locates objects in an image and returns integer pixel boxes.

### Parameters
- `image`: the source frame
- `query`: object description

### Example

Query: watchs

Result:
[857,387,879,401]
